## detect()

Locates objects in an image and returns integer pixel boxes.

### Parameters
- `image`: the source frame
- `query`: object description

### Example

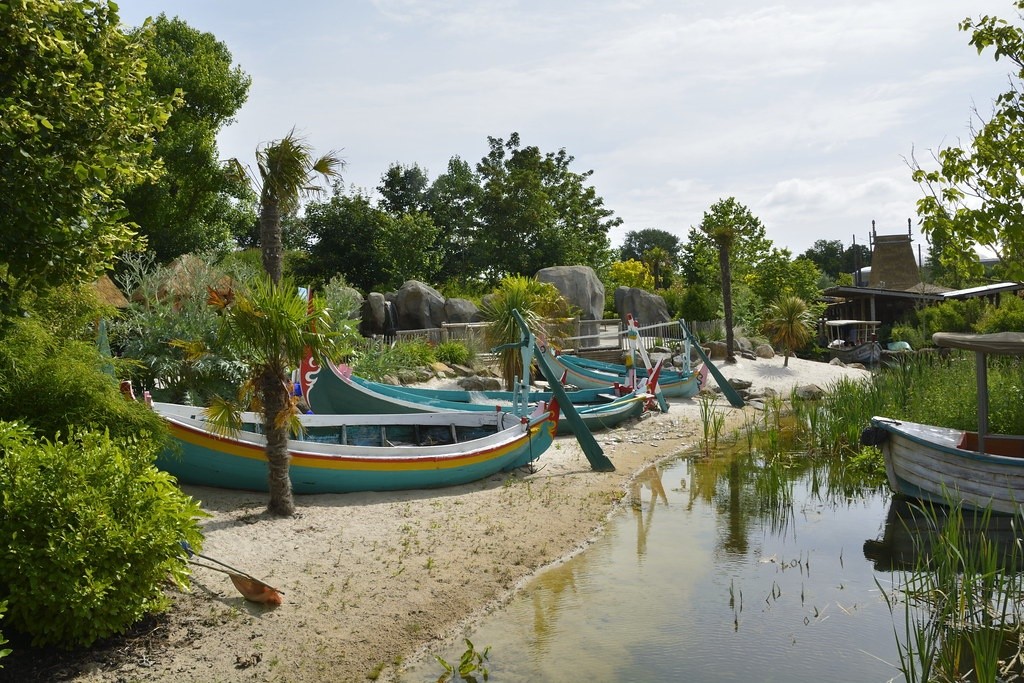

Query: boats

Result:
[825,319,883,364]
[98,283,712,497]
[861,330,1024,520]
